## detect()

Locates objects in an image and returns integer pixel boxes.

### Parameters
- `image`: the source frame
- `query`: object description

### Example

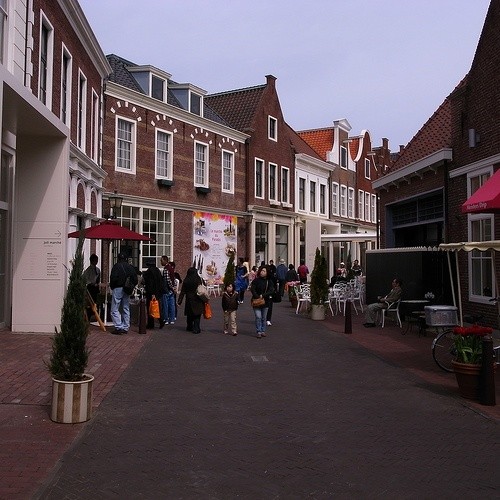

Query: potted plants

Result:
[308,247,330,321]
[44,242,95,424]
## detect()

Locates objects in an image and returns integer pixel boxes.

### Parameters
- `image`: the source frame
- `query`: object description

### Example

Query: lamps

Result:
[468,129,481,148]
[103,188,123,220]
[237,208,253,235]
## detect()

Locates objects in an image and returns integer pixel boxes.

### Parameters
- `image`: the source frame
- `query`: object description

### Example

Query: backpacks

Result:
[127,283,146,306]
[149,298,160,318]
[174,278,179,294]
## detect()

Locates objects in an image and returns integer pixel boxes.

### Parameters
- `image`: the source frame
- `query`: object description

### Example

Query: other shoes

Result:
[170,321,174,325]
[240,300,244,304]
[165,322,168,324]
[232,331,237,336]
[111,328,122,335]
[121,329,128,334]
[224,331,228,334]
[266,321,272,325]
[256,332,262,337]
[262,332,266,337]
[363,322,376,327]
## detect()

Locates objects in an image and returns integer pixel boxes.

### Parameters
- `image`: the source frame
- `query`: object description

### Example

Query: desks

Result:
[400,298,429,331]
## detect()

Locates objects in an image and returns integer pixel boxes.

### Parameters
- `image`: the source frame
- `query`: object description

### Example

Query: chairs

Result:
[205,281,224,298]
[293,275,365,317]
[376,294,402,329]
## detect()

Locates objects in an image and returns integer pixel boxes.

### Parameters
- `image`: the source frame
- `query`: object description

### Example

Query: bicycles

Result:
[431,295,500,374]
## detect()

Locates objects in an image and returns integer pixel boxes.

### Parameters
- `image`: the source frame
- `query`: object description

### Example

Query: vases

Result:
[452,362,500,399]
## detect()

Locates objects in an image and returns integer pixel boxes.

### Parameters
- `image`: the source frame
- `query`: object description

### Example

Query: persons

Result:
[85,253,363,339]
[362,279,403,327]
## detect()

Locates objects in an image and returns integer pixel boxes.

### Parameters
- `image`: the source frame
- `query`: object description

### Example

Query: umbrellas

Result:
[68,220,154,324]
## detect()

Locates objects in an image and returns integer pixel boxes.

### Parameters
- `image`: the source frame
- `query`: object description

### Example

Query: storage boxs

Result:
[425,306,458,327]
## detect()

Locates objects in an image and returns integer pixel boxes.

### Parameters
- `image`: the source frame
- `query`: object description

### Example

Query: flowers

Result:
[425,292,435,301]
[454,323,493,364]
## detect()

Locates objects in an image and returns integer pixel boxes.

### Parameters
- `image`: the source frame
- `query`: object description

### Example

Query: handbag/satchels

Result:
[123,275,135,295]
[196,277,211,299]
[273,291,281,302]
[251,298,265,306]
[203,303,212,319]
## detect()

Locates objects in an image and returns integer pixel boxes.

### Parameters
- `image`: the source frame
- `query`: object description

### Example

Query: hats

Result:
[289,264,294,269]
[340,263,344,265]
[279,258,285,264]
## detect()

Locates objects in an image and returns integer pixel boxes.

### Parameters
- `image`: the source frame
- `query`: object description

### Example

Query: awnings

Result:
[463,170,500,213]
